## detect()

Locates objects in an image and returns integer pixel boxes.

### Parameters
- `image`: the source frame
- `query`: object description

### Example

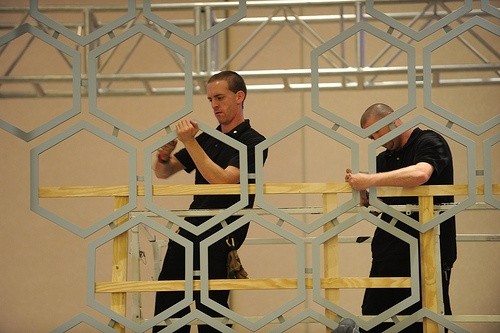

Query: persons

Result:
[152,71,268,333]
[345,104,458,332]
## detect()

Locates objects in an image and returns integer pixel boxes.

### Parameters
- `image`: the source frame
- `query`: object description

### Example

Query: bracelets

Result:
[158,152,170,163]
